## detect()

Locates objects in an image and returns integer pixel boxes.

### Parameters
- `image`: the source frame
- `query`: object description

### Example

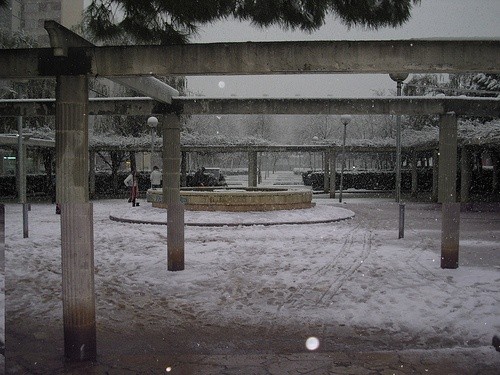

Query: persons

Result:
[123,167,229,208]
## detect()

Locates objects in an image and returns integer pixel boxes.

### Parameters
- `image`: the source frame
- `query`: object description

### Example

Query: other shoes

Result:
[128,197,132,202]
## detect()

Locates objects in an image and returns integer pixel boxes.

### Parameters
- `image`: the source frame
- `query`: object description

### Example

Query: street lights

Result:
[147,116,158,173]
[388,71,410,202]
[312,136,319,173]
[338,114,351,202]
